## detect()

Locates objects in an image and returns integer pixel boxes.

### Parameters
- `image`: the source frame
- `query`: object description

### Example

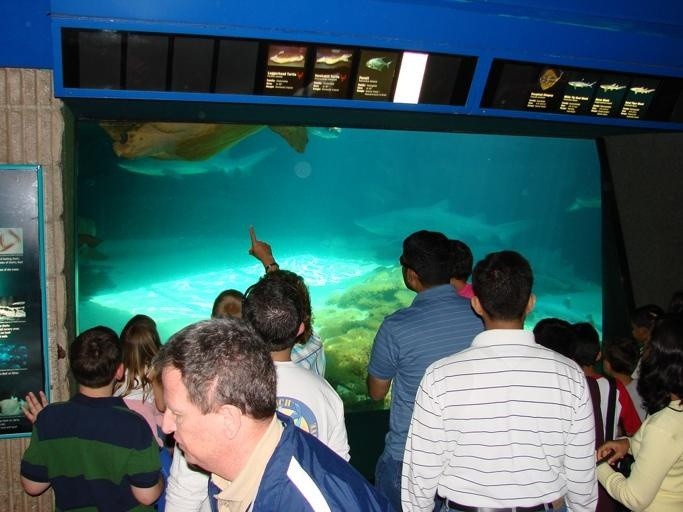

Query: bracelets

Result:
[263,262,279,270]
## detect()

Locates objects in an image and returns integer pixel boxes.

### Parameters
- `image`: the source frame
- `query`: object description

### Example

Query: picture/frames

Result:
[0,163,51,440]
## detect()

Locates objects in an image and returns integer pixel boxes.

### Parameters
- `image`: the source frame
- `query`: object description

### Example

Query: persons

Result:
[237,270,350,467]
[447,239,475,301]
[533,304,683,512]
[397,249,599,511]
[208,222,326,378]
[161,270,351,380]
[148,319,395,512]
[366,229,485,510]
[165,271,349,512]
[17,312,176,512]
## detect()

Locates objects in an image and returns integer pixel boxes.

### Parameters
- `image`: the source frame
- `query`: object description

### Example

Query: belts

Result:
[447,499,553,512]
